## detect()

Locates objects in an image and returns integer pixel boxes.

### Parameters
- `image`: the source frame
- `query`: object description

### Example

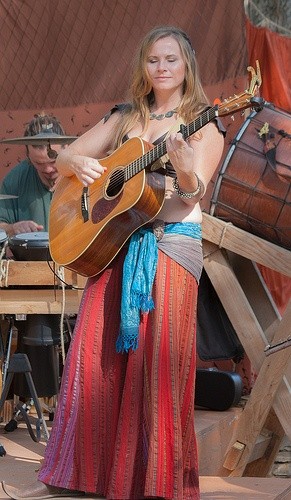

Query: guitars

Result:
[48,89,265,279]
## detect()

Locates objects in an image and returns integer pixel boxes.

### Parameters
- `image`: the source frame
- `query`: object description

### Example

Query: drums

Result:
[0,228,9,260]
[8,231,69,346]
[208,96,291,251]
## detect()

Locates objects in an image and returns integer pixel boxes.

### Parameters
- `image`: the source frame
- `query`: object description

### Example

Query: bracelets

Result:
[172,171,201,199]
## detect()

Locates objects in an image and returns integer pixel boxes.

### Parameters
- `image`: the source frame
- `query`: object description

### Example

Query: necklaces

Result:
[150,105,179,120]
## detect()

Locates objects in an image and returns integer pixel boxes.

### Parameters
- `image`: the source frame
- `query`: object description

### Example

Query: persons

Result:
[38,26,226,500]
[0,114,71,237]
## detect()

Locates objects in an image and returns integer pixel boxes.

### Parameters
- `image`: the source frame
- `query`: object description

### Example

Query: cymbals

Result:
[0,194,19,200]
[0,132,79,145]
